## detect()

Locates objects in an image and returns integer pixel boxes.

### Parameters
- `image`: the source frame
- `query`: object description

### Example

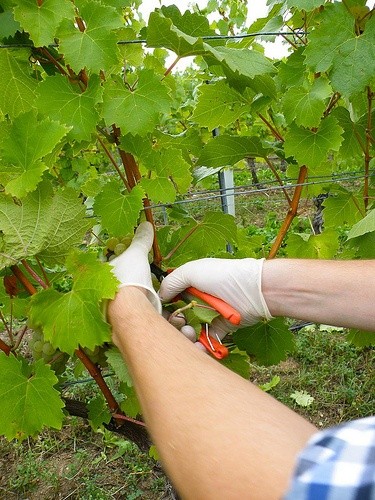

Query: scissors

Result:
[148,261,241,360]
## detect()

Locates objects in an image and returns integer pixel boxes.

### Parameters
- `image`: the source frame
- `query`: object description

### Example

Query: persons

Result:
[95,219,375,500]
